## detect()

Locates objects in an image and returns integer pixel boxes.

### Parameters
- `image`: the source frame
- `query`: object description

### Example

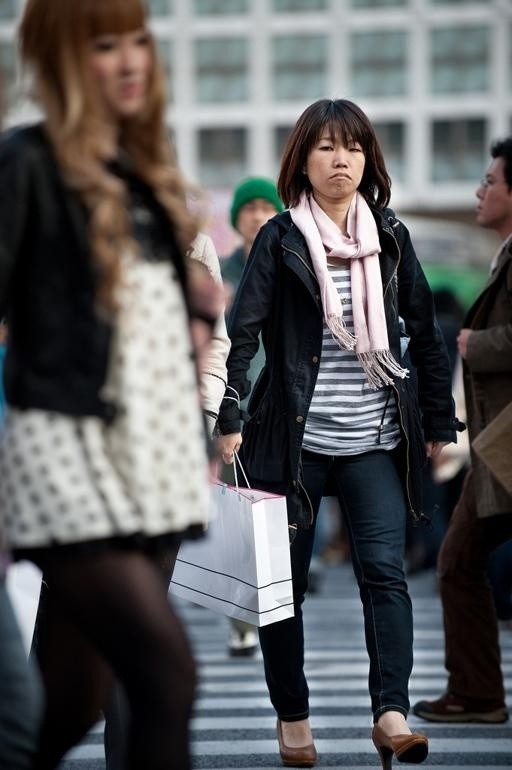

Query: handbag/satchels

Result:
[165,447,298,626]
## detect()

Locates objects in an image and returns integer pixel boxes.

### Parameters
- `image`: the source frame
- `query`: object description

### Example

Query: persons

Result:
[295,279,512,635]
[2,3,217,770]
[213,171,288,657]
[216,96,468,769]
[408,135,512,726]
[180,226,233,494]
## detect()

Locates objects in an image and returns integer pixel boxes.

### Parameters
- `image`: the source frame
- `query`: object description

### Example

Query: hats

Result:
[228,177,284,231]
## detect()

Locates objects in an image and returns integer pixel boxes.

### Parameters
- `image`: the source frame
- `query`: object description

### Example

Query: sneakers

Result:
[413,690,509,723]
[223,628,261,655]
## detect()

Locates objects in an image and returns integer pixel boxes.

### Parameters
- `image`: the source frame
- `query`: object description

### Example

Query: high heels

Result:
[370,720,431,770]
[275,717,318,742]
